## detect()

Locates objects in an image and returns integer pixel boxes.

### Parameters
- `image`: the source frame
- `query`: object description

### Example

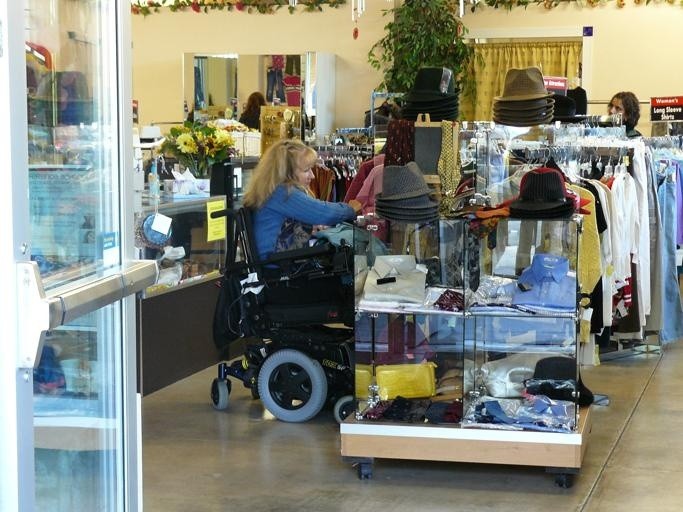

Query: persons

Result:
[238,92,266,132]
[242,139,363,304]
[605,92,642,139]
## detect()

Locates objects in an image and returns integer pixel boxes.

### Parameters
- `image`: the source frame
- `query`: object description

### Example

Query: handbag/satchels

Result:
[212,213,245,348]
[481,354,551,398]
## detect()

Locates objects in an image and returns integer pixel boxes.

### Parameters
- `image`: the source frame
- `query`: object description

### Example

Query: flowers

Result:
[154,120,241,177]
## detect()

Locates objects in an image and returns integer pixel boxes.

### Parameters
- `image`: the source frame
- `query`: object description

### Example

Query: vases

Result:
[196,178,209,194]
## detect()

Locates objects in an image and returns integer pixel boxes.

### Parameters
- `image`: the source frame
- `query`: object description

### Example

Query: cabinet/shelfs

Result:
[5,1,162,511]
[240,51,336,133]
[139,192,229,398]
[339,213,594,489]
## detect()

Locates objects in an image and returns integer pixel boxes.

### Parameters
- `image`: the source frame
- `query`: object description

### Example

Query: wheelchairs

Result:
[209,158,355,424]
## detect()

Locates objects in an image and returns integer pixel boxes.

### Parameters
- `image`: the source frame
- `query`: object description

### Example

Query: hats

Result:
[399,67,460,124]
[491,68,554,126]
[523,356,593,407]
[520,168,592,215]
[374,161,441,225]
[551,94,588,123]
[139,125,165,149]
[33,70,98,128]
[509,173,574,220]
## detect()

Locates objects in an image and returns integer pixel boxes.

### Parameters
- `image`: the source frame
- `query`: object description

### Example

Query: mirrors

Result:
[183,53,239,117]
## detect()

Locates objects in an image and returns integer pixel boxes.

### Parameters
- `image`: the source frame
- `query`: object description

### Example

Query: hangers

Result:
[315,145,364,180]
[524,147,629,172]
[336,127,363,137]
[582,115,624,127]
